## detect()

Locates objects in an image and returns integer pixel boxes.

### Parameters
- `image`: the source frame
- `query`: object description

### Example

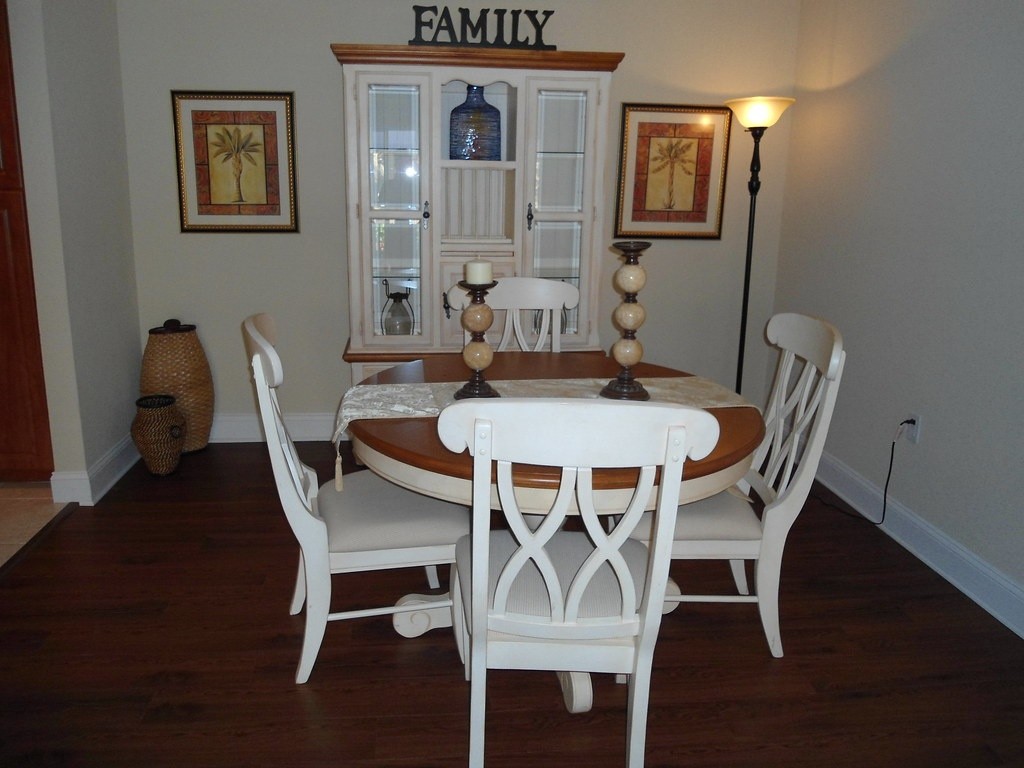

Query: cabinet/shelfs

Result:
[327,42,624,466]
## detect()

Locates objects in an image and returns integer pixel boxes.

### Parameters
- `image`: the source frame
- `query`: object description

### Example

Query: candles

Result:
[465,259,492,284]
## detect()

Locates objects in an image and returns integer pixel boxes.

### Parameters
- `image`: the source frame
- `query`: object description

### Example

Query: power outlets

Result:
[905,413,922,444]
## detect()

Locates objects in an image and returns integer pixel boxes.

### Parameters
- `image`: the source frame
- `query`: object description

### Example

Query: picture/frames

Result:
[612,103,733,241]
[170,89,300,234]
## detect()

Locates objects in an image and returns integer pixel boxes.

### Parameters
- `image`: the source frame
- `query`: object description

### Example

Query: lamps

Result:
[727,96,797,395]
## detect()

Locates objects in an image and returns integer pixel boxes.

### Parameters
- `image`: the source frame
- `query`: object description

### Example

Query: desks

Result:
[347,349,766,714]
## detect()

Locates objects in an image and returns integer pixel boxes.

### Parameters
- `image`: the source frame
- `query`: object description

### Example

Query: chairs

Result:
[446,277,579,353]
[607,312,847,657]
[243,313,472,685]
[437,397,720,768]
